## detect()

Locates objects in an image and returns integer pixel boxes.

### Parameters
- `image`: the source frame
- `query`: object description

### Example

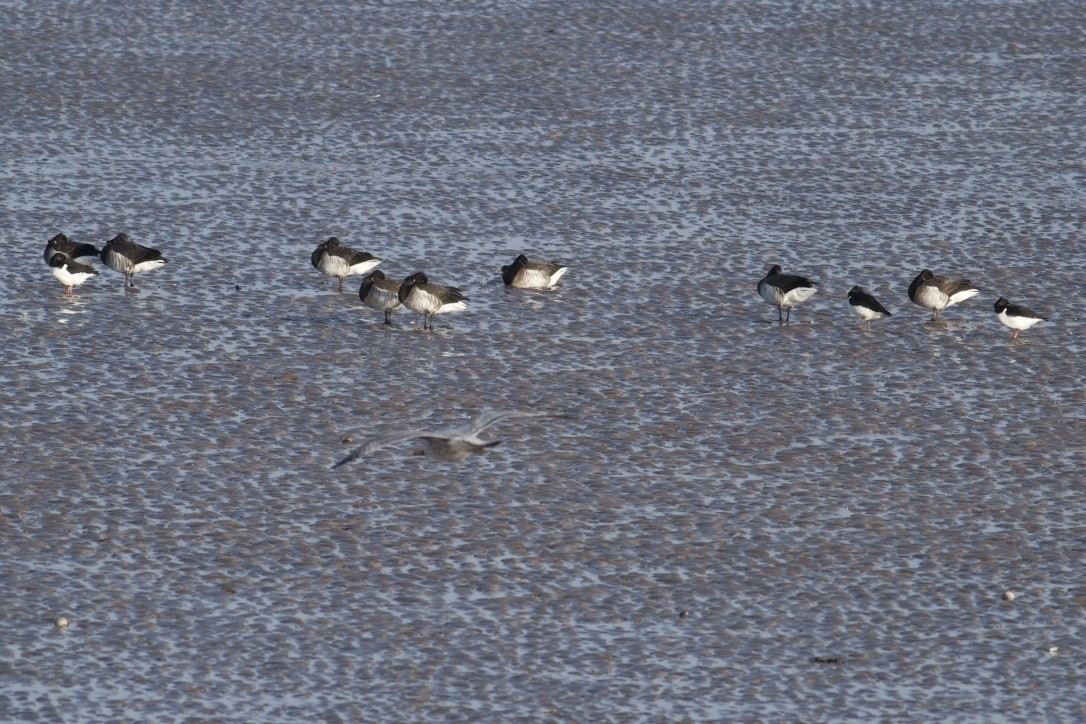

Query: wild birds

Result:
[44,232,170,294]
[847,285,891,330]
[501,254,570,287]
[359,269,402,324]
[757,264,819,322]
[908,269,982,320]
[311,236,384,289]
[398,271,470,328]
[994,297,1049,338]
